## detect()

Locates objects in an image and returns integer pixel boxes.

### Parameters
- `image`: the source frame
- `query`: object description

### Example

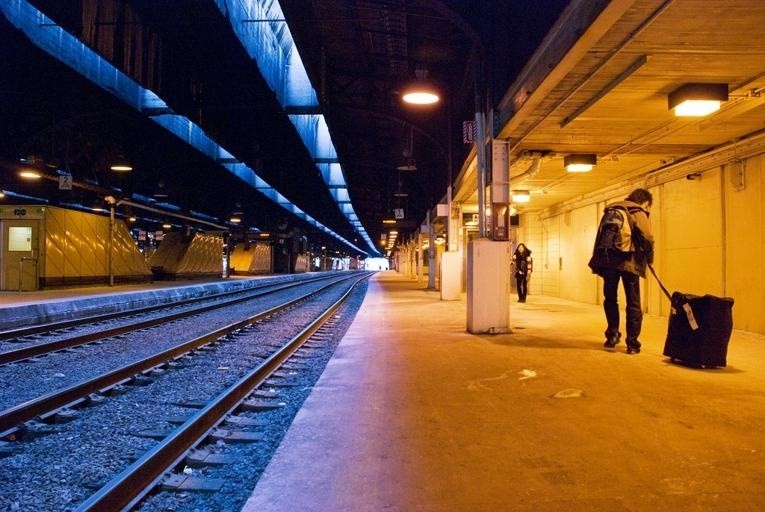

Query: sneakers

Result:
[604,330,621,348]
[627,345,640,354]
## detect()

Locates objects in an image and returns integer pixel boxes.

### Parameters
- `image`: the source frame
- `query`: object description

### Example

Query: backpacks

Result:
[597,205,650,256]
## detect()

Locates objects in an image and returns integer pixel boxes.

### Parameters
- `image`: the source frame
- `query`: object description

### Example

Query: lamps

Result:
[667,81,731,117]
[563,156,597,172]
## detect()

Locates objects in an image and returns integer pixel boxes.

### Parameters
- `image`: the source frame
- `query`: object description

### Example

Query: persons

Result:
[586,187,656,354]
[509,242,534,303]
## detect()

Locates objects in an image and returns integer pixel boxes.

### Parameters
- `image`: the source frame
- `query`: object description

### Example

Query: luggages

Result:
[662,291,735,368]
